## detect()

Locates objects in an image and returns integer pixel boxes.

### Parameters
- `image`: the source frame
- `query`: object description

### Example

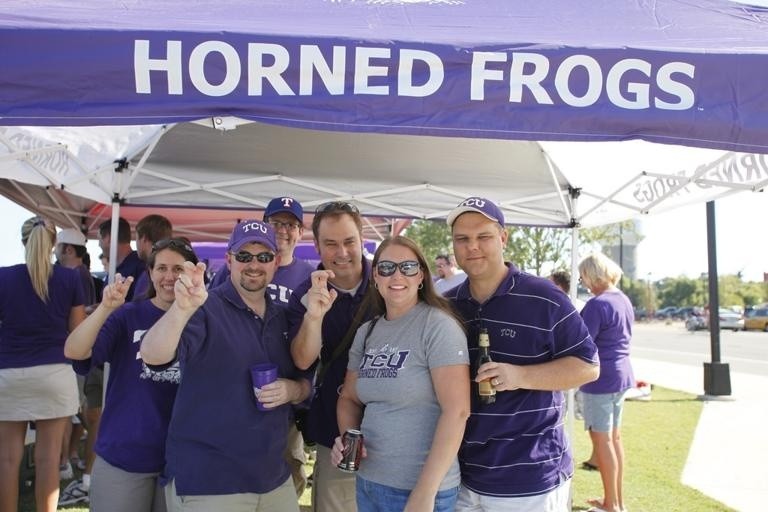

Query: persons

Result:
[0,195,601,512]
[574,252,637,511]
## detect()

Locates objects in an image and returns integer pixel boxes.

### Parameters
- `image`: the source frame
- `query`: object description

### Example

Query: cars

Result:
[632,303,768,334]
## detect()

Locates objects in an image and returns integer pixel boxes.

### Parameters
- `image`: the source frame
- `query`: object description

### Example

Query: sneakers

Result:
[58,479,90,506]
[70,454,87,470]
[579,460,600,470]
[584,497,627,512]
[59,462,73,479]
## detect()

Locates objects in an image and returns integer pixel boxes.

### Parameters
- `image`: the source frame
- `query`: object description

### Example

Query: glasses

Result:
[150,238,193,254]
[314,200,361,216]
[266,219,301,232]
[372,259,420,278]
[228,250,277,265]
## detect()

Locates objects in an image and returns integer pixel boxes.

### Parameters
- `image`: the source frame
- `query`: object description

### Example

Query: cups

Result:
[249,362,277,412]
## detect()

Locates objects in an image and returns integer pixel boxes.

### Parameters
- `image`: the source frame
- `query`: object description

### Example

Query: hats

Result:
[263,196,304,224]
[445,196,506,228]
[54,227,88,247]
[227,219,278,254]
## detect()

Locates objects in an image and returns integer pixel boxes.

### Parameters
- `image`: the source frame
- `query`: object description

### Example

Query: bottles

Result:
[474,326,500,407]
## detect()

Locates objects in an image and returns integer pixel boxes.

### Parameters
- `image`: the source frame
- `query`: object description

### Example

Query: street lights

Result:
[648,270,652,319]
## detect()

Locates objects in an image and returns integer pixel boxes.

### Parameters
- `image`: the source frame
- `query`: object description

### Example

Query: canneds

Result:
[335,428,363,472]
